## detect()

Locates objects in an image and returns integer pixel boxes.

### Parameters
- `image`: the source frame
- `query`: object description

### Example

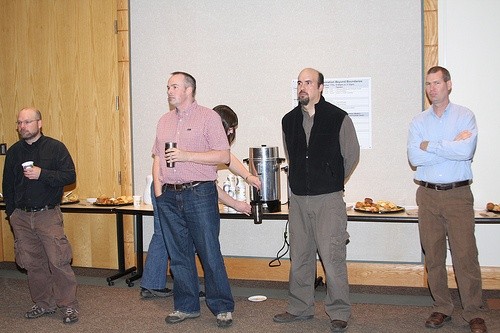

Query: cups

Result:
[222,173,246,212]
[22,161,33,172]
[165,142,176,168]
[133,196,141,207]
[250,200,262,224]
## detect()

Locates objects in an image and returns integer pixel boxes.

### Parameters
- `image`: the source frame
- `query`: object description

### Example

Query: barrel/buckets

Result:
[243,144,285,214]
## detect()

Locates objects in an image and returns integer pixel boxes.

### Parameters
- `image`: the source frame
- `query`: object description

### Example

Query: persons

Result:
[273,68,360,332]
[138,105,262,299]
[152,72,235,327]
[407,66,487,333]
[2,107,78,322]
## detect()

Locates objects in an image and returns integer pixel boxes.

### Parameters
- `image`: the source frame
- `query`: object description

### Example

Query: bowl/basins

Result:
[87,198,97,204]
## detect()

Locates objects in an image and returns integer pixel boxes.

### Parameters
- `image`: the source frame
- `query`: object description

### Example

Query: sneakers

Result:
[25,304,55,318]
[166,308,200,324]
[63,307,78,323]
[140,286,173,298]
[216,310,232,328]
[198,290,205,300]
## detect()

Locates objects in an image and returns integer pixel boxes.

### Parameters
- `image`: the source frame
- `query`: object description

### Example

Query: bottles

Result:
[143,175,152,204]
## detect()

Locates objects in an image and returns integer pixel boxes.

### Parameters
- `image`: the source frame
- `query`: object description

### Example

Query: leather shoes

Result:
[425,312,451,328]
[470,317,486,333]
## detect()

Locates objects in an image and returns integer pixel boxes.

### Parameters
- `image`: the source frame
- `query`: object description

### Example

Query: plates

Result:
[488,209,500,214]
[61,200,80,205]
[93,202,133,206]
[248,295,268,302]
[354,205,405,214]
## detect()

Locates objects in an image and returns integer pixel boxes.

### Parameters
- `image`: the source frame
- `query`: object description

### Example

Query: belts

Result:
[22,204,55,212]
[420,180,469,191]
[166,180,203,190]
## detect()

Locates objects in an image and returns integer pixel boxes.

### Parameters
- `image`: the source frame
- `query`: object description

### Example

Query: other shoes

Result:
[273,313,313,322]
[332,319,346,332]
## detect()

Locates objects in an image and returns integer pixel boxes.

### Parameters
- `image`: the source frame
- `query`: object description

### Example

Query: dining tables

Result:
[0,199,500,294]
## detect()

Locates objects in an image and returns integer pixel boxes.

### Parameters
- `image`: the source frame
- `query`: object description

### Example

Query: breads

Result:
[355,197,398,212]
[62,195,79,202]
[95,195,134,205]
[487,202,500,211]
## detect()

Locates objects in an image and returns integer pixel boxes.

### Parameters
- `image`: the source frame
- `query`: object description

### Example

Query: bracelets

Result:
[245,175,253,180]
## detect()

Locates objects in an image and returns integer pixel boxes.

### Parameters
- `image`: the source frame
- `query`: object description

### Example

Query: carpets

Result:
[0,278,500,333]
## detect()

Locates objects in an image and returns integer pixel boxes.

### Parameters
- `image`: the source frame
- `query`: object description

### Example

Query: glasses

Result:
[16,119,41,126]
